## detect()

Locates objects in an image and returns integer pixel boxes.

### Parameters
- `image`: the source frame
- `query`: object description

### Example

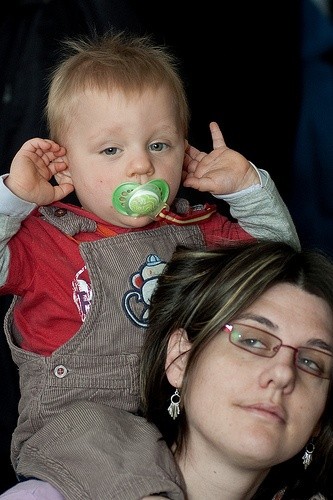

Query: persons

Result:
[0,239,333,500]
[0,28,333,500]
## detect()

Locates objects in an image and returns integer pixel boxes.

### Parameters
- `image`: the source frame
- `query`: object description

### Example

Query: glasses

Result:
[225,323,333,380]
